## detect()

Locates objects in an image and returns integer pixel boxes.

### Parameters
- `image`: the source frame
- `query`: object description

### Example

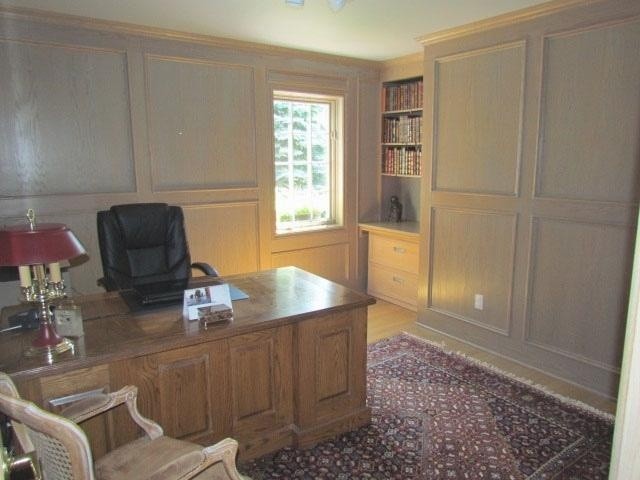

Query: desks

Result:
[1,265,378,469]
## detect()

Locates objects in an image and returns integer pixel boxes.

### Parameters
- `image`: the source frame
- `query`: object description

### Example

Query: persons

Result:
[187,289,211,306]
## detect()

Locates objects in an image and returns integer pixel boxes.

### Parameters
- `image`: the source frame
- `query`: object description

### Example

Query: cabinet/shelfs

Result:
[379,77,423,178]
[366,232,418,312]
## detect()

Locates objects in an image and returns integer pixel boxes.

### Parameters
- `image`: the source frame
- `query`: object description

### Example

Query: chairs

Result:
[1,373,250,480]
[96,202,218,291]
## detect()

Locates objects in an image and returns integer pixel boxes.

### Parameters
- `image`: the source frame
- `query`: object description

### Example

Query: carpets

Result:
[239,330,614,478]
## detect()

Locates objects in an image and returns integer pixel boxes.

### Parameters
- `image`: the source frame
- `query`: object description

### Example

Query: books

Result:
[381,80,424,175]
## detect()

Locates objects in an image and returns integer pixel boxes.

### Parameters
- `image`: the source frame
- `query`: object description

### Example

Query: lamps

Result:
[0,207,88,365]
